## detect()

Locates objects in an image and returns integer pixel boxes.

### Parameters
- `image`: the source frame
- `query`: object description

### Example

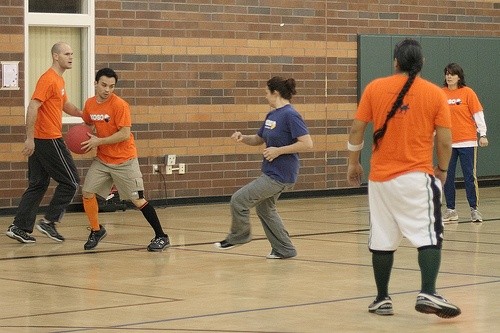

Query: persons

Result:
[80,68,170,252]
[214,75,314,258]
[6,42,83,243]
[346,39,462,319]
[434,63,488,223]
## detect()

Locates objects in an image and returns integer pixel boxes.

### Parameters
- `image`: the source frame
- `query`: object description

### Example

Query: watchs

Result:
[480,135,487,138]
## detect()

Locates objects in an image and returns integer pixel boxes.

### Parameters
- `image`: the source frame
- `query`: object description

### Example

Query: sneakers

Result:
[215,240,236,250]
[35,219,65,242]
[470,207,483,222]
[266,251,282,258]
[368,295,393,314]
[441,209,459,221]
[5,223,37,243]
[83,225,107,250]
[146,234,170,252]
[415,292,462,319]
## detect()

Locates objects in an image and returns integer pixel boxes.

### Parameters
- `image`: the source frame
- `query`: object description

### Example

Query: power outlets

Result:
[165,154,176,165]
[179,163,185,174]
[152,164,158,175]
[166,165,172,174]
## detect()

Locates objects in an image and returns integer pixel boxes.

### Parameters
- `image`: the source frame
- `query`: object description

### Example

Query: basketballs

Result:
[65,125,94,154]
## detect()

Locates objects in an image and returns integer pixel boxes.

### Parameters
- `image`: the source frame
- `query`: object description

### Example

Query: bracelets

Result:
[437,164,448,172]
[348,140,364,151]
